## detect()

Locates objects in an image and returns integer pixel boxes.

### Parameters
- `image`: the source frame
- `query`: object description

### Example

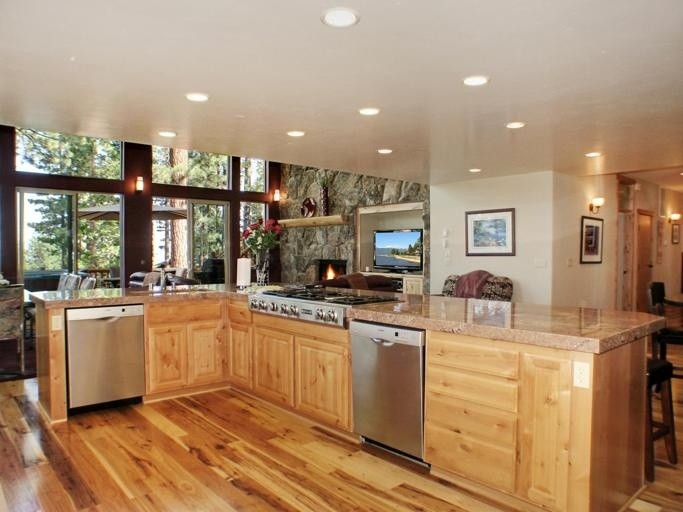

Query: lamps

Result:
[589,198,604,214]
[135,175,144,193]
[273,189,281,204]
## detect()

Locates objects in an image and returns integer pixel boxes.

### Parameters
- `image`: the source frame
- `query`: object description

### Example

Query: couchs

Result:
[304,272,395,292]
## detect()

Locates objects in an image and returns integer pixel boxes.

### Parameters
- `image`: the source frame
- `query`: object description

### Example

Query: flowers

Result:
[242,218,282,269]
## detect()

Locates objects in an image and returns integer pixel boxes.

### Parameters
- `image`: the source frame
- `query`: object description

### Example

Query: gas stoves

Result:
[246,283,399,330]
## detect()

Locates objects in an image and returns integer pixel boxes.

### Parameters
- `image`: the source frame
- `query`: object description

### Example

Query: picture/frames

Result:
[580,216,604,263]
[465,207,516,256]
[671,224,680,244]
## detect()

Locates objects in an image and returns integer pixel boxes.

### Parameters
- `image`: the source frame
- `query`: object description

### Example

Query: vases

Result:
[255,269,266,286]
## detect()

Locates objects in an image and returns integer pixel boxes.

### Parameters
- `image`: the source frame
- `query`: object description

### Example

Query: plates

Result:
[299,196,316,219]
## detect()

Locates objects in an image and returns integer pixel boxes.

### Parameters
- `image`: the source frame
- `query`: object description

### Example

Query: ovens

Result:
[61,305,147,415]
[345,316,431,471]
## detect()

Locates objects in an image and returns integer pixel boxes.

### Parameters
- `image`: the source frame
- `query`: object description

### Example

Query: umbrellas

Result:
[77,204,193,222]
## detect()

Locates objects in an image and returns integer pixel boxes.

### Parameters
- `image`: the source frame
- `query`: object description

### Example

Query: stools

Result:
[645,357,676,482]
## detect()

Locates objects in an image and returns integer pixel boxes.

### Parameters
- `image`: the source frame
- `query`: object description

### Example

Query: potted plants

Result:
[315,169,339,216]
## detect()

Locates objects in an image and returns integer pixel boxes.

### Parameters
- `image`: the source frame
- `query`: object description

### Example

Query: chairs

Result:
[430,270,513,301]
[647,281,683,391]
[0,271,202,375]
